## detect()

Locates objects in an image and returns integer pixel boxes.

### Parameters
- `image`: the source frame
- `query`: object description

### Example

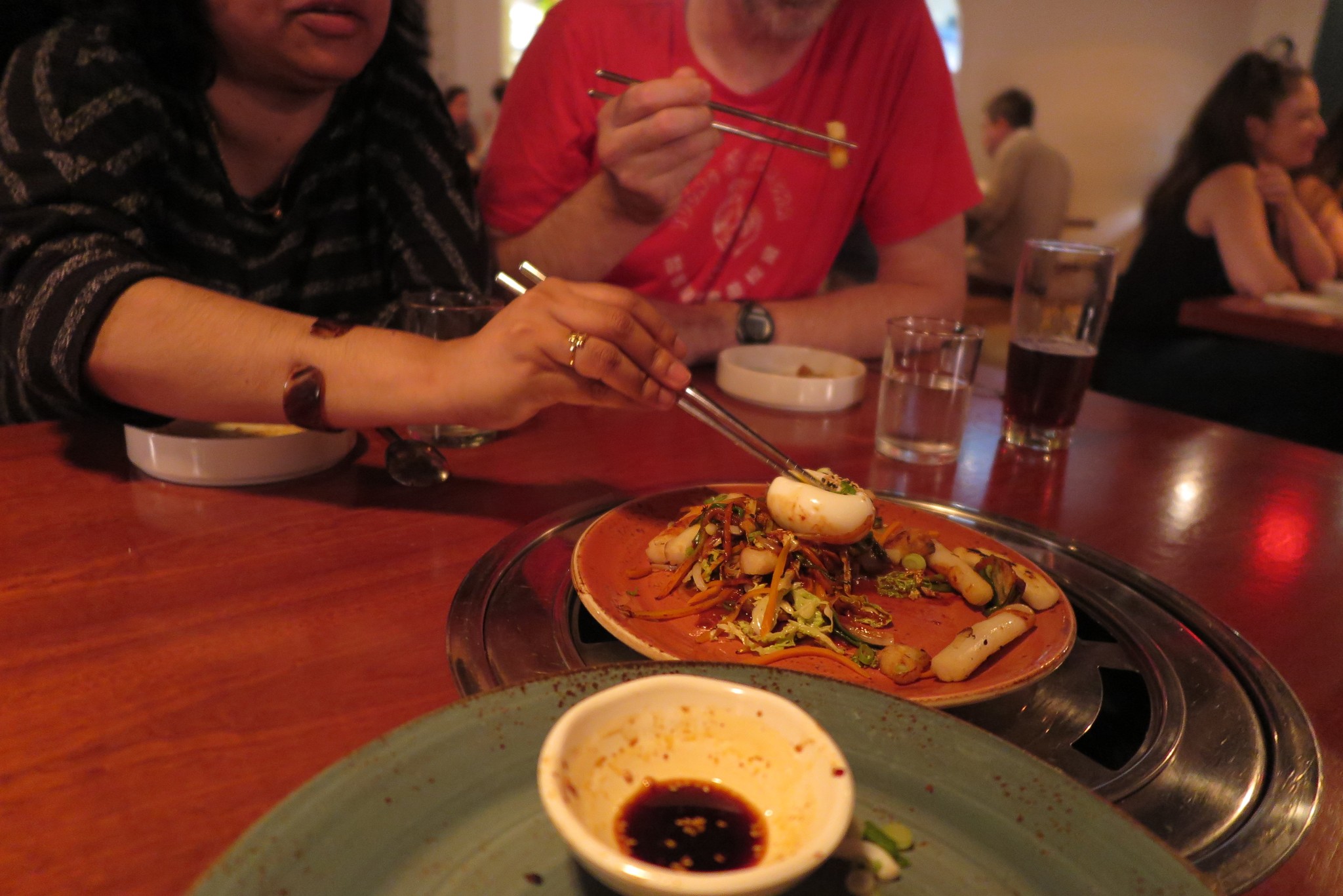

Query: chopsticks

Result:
[496,256,837,490]
[586,66,859,158]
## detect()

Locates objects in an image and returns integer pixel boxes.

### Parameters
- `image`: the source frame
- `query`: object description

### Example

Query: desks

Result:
[1179,297,1343,349]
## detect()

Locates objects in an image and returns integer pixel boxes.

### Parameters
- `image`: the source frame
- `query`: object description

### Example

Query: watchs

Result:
[734,298,775,345]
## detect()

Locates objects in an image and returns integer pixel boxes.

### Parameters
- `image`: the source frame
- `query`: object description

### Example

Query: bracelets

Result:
[281,312,358,432]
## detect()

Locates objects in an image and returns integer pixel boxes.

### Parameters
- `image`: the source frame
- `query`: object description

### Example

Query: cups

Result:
[873,316,984,467]
[995,236,1119,453]
[400,285,505,449]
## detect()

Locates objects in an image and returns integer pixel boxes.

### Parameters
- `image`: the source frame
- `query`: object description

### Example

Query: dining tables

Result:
[0,353,1343,896]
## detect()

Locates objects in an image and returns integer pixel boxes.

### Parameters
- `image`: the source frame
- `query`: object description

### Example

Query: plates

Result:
[716,339,868,414]
[572,478,1078,714]
[537,674,855,895]
[124,406,358,485]
[177,660,1229,895]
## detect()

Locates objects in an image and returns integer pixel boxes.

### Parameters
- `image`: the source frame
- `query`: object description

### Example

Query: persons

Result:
[965,87,1074,301]
[442,77,509,172]
[3,1,697,434]
[1094,33,1341,453]
[474,0,986,373]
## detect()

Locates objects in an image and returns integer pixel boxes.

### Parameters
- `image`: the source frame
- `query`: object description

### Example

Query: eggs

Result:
[766,468,875,544]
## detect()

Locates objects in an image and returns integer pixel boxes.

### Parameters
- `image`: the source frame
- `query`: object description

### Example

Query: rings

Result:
[567,330,590,371]
